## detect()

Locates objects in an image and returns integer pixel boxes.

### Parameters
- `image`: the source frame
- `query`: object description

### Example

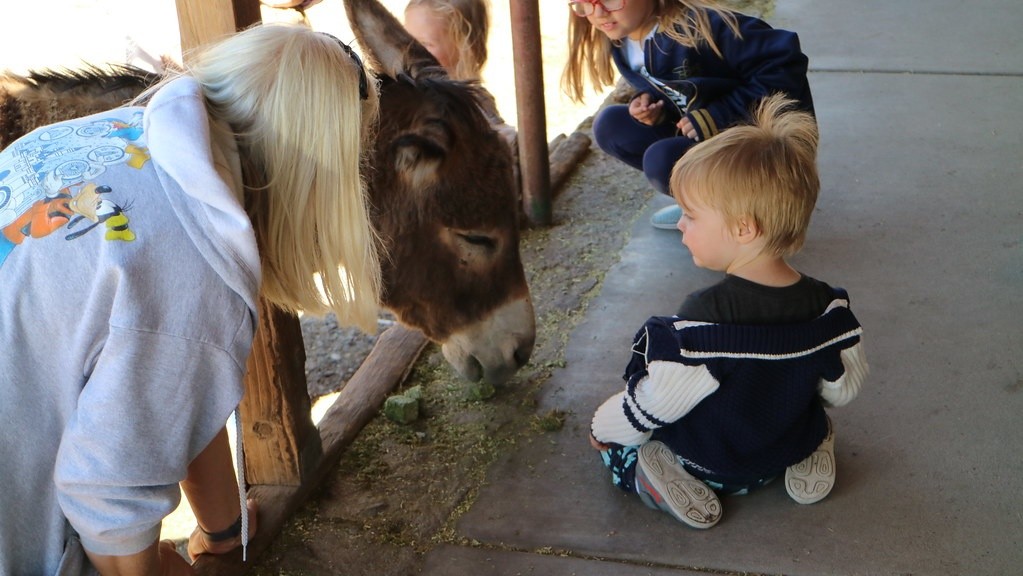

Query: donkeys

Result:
[0,0,537,382]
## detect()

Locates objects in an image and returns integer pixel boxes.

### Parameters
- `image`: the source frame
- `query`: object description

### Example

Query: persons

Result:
[589,90,868,530]
[559,0,817,230]
[0,24,382,576]
[402,0,521,200]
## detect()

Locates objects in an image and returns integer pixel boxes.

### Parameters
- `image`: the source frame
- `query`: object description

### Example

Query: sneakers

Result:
[650,204,681,229]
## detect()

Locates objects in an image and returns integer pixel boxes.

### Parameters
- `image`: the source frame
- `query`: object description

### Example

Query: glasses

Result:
[568,0,625,17]
[318,31,369,101]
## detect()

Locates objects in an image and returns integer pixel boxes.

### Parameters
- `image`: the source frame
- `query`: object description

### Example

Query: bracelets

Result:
[196,515,242,542]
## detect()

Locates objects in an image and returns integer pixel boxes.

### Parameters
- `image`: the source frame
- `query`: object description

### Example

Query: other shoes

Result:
[634,440,723,529]
[784,414,835,504]
